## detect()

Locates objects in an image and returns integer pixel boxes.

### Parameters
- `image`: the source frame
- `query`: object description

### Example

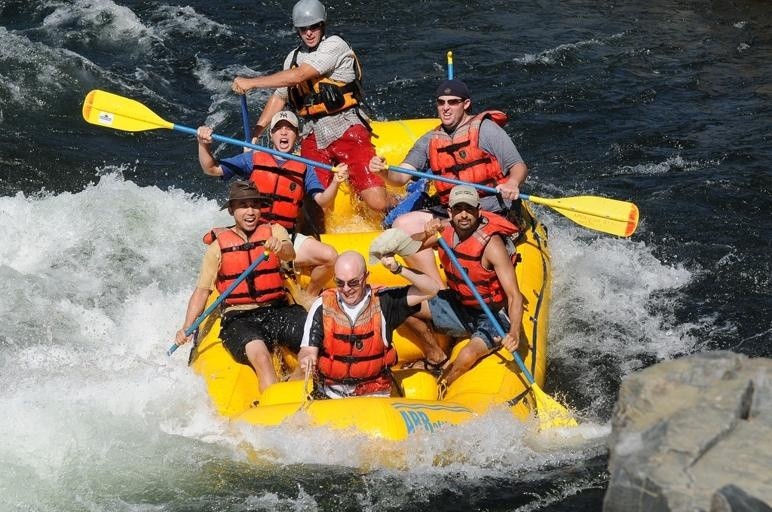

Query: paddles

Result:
[82,88,343,182]
[435,232,579,431]
[380,165,639,237]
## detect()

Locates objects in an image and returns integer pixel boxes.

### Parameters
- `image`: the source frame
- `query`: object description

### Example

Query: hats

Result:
[449,182,480,209]
[219,180,273,211]
[269,110,300,130]
[369,228,423,266]
[437,79,472,112]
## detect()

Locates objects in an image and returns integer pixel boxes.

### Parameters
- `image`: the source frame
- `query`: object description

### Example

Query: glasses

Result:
[333,274,365,287]
[437,98,463,105]
[298,23,322,32]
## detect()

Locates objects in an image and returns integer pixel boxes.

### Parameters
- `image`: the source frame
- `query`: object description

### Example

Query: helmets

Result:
[292,0,328,27]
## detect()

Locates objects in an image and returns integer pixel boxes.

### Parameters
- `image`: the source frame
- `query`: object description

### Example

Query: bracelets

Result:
[391,261,402,274]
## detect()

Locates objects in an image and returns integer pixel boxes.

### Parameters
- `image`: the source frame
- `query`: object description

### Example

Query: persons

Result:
[174,180,308,394]
[230,0,398,215]
[298,249,440,401]
[369,79,528,289]
[197,110,350,304]
[404,185,524,399]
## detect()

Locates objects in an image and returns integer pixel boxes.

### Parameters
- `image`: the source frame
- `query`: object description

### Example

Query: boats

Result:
[182,117,560,466]
